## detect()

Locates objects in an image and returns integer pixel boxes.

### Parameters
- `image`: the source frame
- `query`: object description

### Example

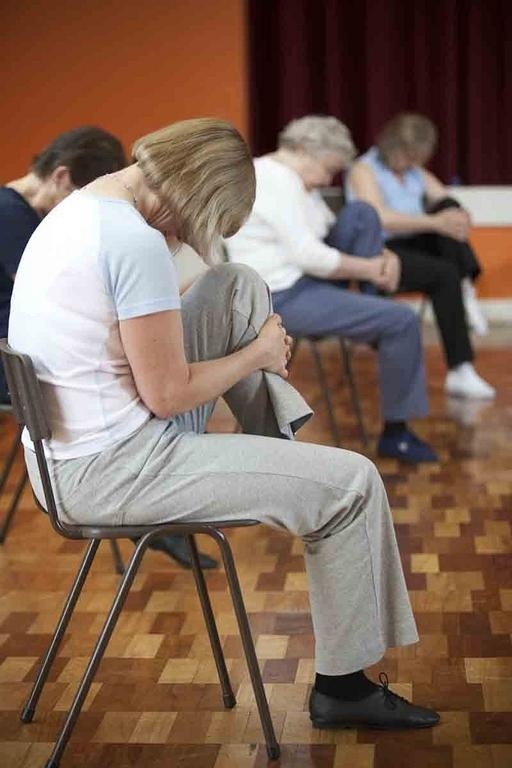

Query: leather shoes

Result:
[131,535,219,569]
[308,676,440,730]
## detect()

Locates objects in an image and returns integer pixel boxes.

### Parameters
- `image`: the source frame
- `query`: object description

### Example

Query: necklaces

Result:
[106,173,138,211]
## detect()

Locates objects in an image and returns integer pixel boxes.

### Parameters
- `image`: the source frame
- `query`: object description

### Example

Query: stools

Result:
[233,332,368,450]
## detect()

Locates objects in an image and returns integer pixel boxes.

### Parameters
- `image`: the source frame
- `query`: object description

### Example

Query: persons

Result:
[1,125,125,401]
[345,113,495,399]
[8,116,445,731]
[217,115,442,463]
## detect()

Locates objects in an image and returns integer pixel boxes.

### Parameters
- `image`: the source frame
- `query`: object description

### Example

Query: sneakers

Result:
[377,430,438,463]
[460,276,489,338]
[443,361,496,400]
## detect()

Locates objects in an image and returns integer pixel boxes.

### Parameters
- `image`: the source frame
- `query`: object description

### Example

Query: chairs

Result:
[316,188,428,322]
[1,336,284,767]
